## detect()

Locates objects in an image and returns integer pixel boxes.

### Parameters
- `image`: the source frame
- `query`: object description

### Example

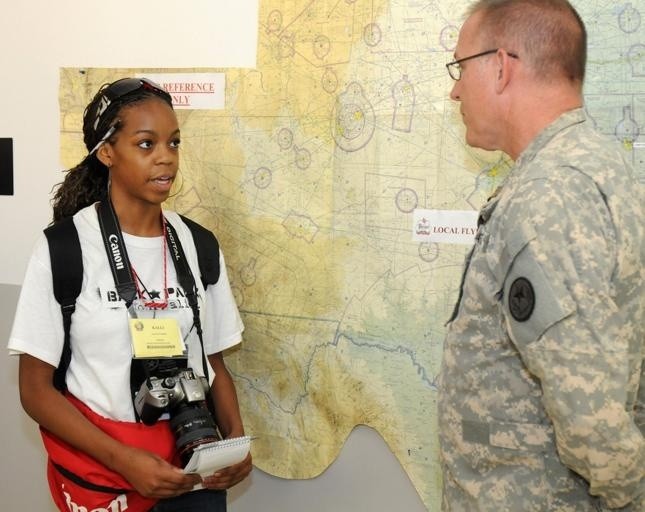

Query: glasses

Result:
[444,49,520,82]
[87,78,173,135]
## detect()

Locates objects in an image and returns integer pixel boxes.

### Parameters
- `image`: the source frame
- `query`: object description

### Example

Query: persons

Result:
[6,77,253,512]
[437,0,644,512]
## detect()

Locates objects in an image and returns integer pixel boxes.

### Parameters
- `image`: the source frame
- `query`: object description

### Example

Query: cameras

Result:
[131,368,221,468]
